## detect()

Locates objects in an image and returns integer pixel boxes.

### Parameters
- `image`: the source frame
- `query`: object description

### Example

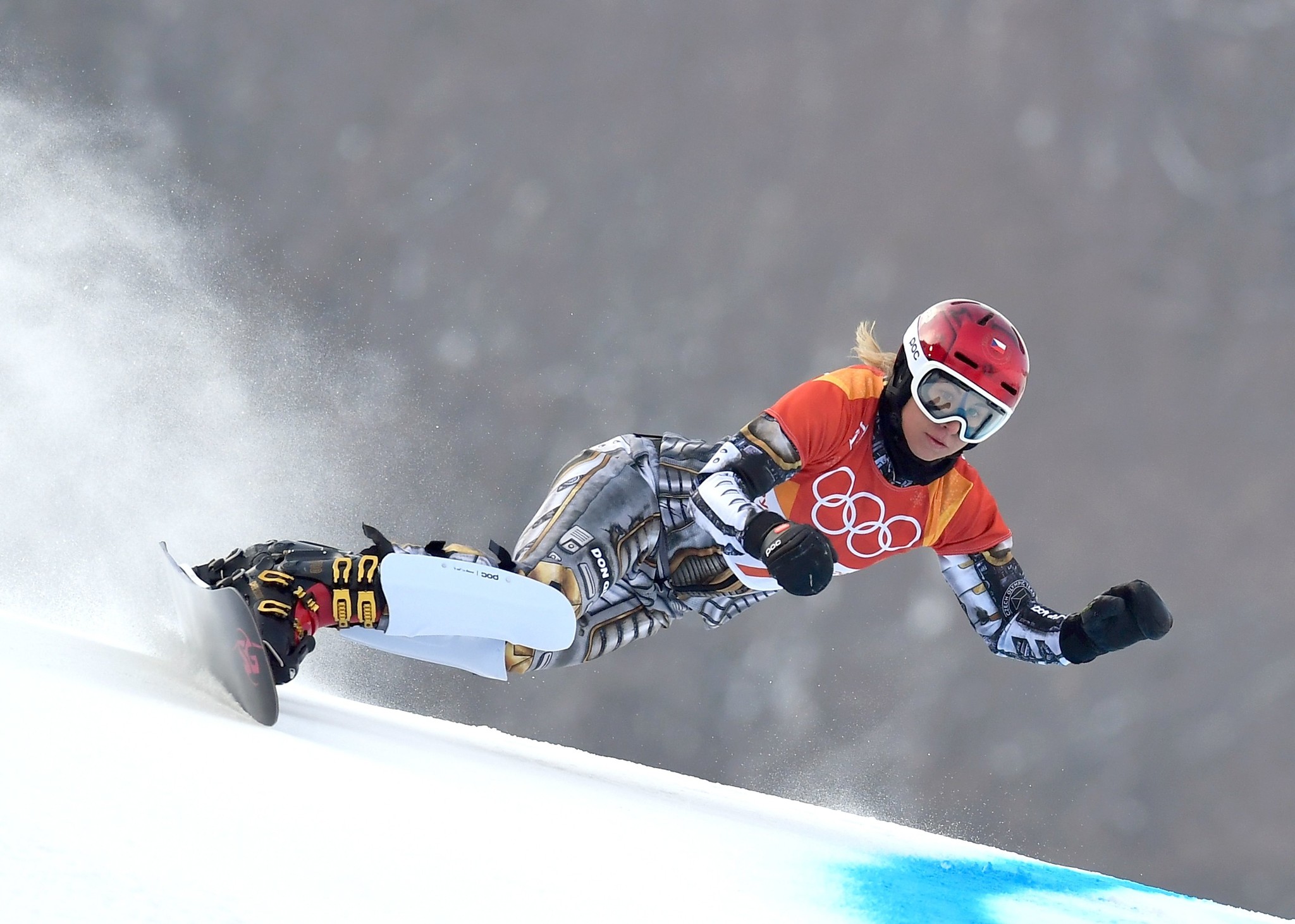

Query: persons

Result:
[192,294,1177,688]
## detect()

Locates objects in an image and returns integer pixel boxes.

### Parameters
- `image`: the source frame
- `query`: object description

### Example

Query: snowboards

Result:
[149,536,278,727]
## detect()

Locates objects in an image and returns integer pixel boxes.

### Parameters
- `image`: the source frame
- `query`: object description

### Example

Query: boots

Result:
[220,558,388,685]
[192,539,378,593]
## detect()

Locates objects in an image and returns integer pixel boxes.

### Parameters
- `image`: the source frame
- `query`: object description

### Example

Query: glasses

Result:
[907,361,1011,444]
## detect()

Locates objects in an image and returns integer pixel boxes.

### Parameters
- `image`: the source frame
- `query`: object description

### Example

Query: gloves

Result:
[1059,580,1173,666]
[742,511,838,598]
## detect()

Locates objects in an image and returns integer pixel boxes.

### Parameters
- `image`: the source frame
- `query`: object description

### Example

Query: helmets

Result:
[902,299,1030,417]
